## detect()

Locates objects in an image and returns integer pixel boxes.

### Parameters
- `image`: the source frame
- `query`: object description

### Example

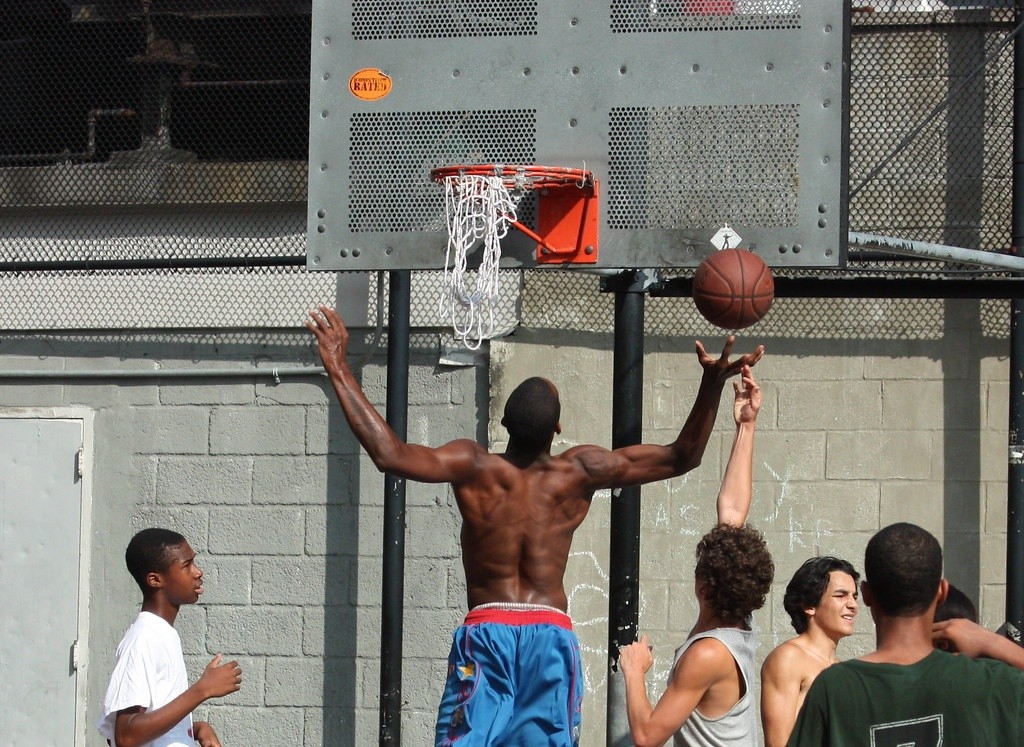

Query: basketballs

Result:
[692,247,774,330]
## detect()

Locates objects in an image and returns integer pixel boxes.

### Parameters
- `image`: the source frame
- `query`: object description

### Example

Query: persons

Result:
[304,302,764,747]
[760,521,1024,747]
[618,364,774,747]
[98,528,242,747]
